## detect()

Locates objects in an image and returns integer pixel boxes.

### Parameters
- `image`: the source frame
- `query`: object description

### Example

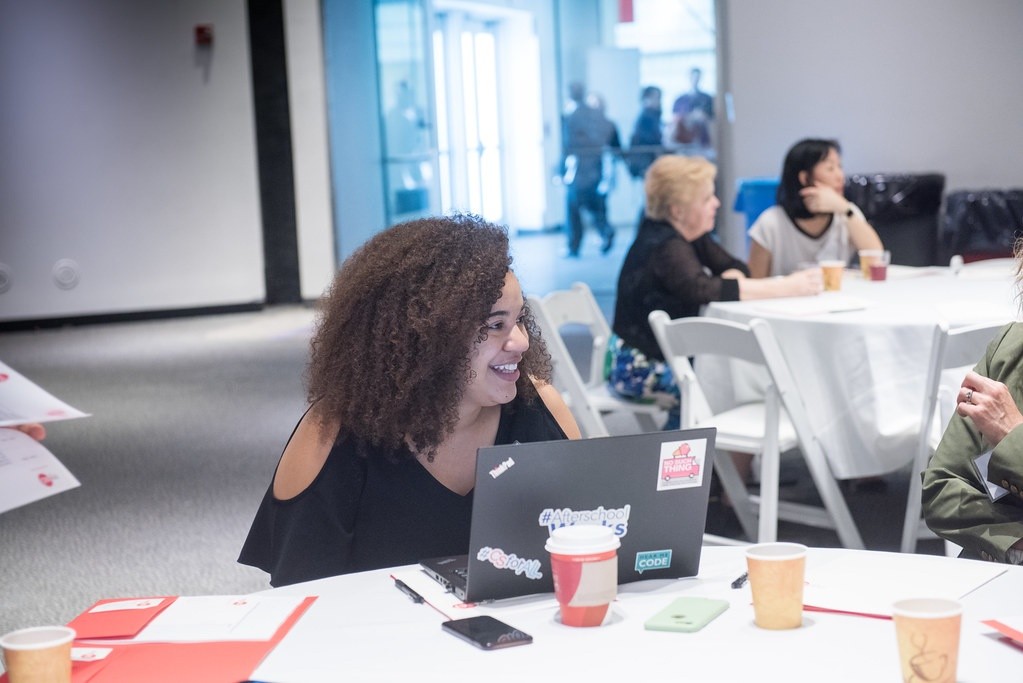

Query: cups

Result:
[890,596,963,683]
[818,260,847,291]
[859,249,889,281]
[0,625,76,683]
[743,541,809,629]
[544,524,621,626]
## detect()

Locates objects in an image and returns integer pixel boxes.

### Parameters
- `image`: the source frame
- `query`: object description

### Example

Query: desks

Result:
[77,533,1023,683]
[692,258,1023,555]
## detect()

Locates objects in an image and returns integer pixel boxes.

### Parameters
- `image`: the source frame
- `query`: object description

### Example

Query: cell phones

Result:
[644,597,731,631]
[442,616,533,650]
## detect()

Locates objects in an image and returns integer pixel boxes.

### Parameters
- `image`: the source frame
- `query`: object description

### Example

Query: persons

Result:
[922,236,1023,568]
[237,218,583,588]
[602,154,825,538]
[746,139,885,277]
[379,80,428,214]
[626,86,669,175]
[671,68,720,150]
[559,82,627,255]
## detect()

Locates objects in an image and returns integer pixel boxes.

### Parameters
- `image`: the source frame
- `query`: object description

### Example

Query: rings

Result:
[966,389,973,402]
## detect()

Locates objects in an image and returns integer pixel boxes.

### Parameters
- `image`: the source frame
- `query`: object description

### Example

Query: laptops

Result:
[420,428,718,603]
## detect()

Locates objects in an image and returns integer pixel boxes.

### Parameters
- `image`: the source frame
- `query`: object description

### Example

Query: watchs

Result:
[842,206,856,220]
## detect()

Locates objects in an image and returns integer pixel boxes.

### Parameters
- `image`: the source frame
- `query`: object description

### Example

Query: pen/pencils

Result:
[390,575,453,622]
[730,570,750,589]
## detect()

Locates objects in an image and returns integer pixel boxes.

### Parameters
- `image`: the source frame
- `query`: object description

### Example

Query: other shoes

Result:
[602,229,615,252]
[569,239,580,256]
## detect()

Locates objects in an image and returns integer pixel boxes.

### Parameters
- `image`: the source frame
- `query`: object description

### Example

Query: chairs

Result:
[524,283,1016,560]
